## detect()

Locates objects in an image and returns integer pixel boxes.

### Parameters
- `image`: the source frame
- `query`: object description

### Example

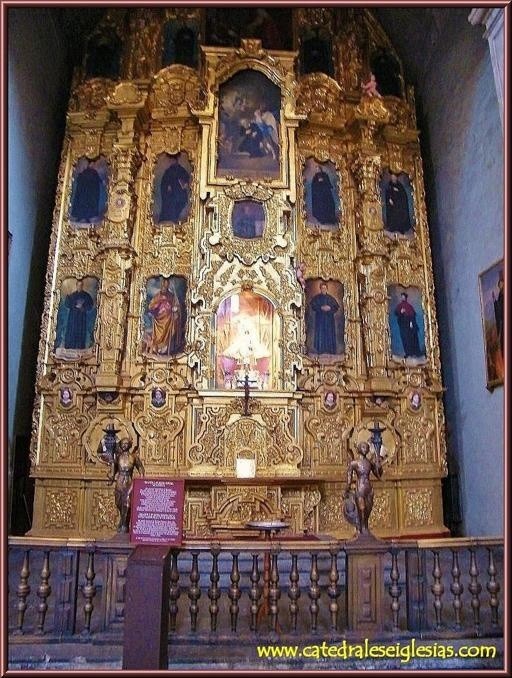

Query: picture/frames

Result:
[478,256,504,389]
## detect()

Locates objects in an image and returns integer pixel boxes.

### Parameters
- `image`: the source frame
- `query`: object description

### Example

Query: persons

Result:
[322,391,337,410]
[346,442,384,534]
[302,28,331,74]
[63,279,95,350]
[385,173,411,234]
[158,156,191,222]
[60,390,73,407]
[309,281,340,354]
[236,204,257,238]
[394,290,422,358]
[217,96,280,161]
[151,387,167,407]
[311,166,335,223]
[490,269,504,363]
[410,393,422,410]
[105,436,145,533]
[69,159,107,223]
[173,20,197,68]
[147,278,182,357]
